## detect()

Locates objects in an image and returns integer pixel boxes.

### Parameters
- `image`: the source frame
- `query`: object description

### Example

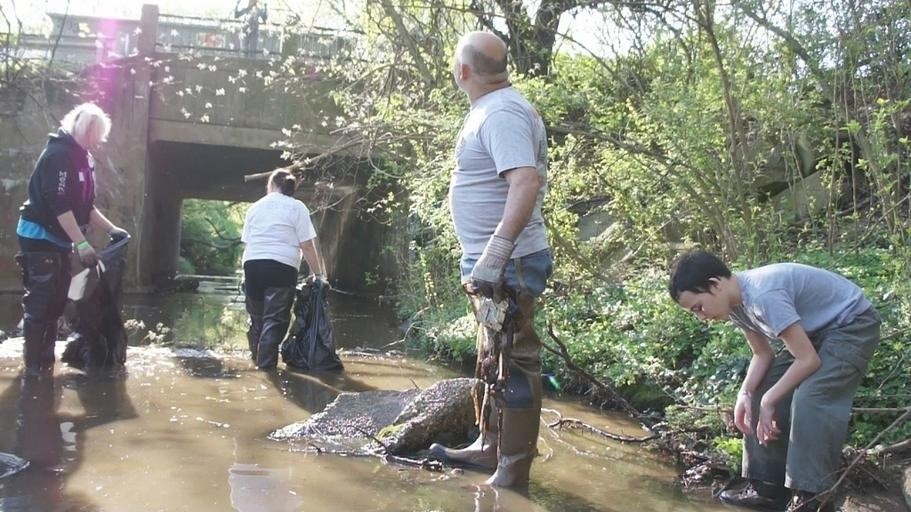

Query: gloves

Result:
[107,226,132,244]
[76,238,103,268]
[470,234,517,304]
[240,279,247,295]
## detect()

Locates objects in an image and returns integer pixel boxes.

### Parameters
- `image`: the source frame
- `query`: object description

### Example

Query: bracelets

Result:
[77,242,88,250]
[738,390,752,398]
[315,274,322,275]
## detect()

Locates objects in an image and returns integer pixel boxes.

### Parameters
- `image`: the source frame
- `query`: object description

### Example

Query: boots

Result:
[24,319,57,371]
[429,396,497,475]
[248,334,278,367]
[482,407,541,488]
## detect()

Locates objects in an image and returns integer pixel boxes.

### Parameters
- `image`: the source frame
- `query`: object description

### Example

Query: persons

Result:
[14,103,129,371]
[428,30,552,489]
[240,168,324,371]
[672,251,882,512]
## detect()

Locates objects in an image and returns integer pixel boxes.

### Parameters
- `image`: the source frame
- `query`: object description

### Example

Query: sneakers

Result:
[784,490,836,511]
[719,480,791,510]
